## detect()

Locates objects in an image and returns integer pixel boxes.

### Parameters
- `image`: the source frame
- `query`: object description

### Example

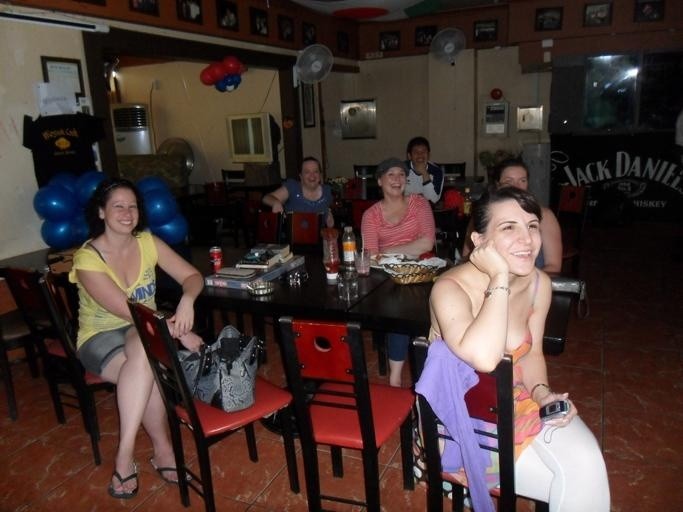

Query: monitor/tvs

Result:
[226,113,281,163]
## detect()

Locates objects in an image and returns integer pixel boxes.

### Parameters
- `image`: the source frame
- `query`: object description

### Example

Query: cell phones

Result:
[539,400,570,421]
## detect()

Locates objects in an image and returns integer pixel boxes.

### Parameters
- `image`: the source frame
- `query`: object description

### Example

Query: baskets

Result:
[383,262,440,285]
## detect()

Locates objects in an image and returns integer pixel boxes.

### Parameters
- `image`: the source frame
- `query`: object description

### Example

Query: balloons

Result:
[33,170,189,251]
[200,55,249,92]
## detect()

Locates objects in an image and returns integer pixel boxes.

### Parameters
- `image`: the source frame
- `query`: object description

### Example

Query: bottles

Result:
[341,226,355,267]
[319,228,339,279]
[463,187,471,216]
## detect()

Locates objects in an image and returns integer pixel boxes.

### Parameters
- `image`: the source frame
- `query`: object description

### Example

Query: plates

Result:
[368,253,416,271]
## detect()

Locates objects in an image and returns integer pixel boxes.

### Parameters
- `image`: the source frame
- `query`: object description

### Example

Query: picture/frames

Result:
[39,53,85,105]
[77,0,667,53]
[299,82,316,129]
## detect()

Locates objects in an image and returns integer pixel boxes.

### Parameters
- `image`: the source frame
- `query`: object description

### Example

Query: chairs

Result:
[278,317,418,512]
[410,336,548,512]
[129,295,302,512]
[0,168,596,466]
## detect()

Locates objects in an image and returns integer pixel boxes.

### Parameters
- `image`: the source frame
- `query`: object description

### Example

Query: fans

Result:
[425,26,465,68]
[287,42,335,89]
[151,137,196,186]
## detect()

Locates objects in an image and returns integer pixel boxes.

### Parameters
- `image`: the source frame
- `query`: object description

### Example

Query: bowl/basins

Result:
[444,174,460,180]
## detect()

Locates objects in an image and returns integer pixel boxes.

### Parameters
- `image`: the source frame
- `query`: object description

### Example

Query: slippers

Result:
[108,456,139,498]
[149,456,192,484]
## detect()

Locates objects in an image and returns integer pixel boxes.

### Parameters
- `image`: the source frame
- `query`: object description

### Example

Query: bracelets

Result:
[482,285,516,299]
[529,381,552,400]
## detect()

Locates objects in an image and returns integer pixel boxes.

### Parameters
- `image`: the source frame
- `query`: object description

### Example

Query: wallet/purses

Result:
[216,267,257,279]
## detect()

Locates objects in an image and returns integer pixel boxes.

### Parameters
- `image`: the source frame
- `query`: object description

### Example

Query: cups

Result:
[353,249,369,278]
[337,266,358,300]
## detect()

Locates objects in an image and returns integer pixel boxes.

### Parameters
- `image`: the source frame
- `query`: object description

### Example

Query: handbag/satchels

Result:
[177,324,263,414]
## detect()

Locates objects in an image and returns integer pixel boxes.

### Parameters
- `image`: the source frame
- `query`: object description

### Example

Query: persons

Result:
[69,175,206,500]
[262,156,336,228]
[403,136,447,204]
[459,158,565,280]
[418,187,612,511]
[359,155,436,387]
